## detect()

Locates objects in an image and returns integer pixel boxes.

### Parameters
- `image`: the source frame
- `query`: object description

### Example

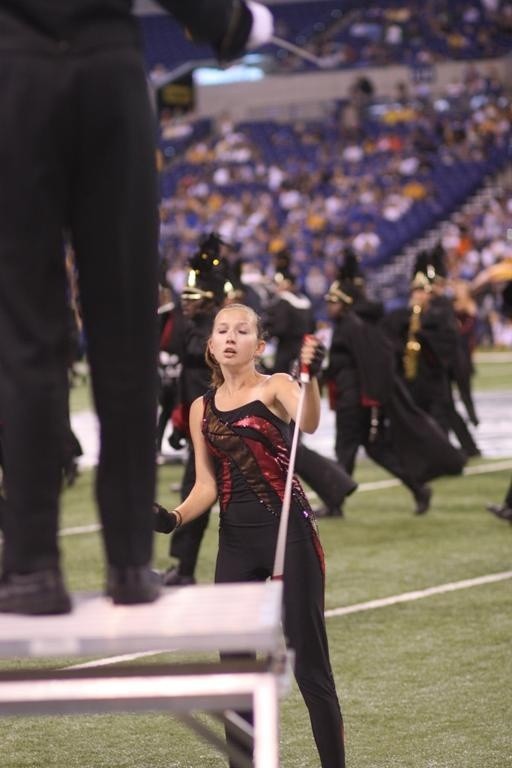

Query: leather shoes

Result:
[106,563,161,603]
[0,566,70,612]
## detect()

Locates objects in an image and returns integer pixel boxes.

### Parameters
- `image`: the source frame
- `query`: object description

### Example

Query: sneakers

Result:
[313,506,341,519]
[415,488,431,515]
[487,502,512,522]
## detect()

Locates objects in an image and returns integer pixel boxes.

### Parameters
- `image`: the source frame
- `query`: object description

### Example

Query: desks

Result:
[0,574,299,766]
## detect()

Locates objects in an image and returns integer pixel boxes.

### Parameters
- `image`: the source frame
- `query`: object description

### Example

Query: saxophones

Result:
[404,300,421,379]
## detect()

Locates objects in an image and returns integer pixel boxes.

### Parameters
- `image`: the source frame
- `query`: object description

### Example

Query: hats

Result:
[323,242,368,305]
[276,245,292,261]
[409,239,447,290]
[181,233,241,307]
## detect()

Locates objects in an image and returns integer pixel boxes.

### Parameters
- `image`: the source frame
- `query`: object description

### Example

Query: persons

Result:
[0,0,274,618]
[150,0,512,583]
[149,303,346,768]
